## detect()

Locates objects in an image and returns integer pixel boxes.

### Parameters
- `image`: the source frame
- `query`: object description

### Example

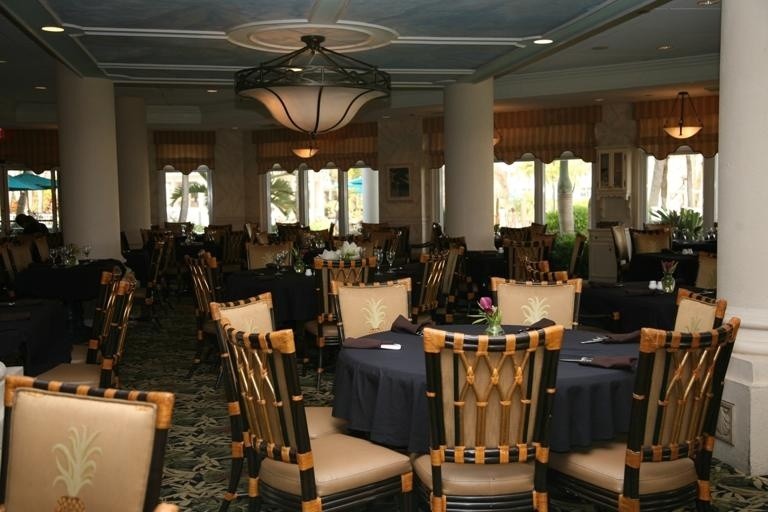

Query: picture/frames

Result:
[384,161,414,204]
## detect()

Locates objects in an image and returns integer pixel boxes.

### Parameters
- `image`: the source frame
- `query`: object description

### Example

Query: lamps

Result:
[231,31,398,141]
[663,86,706,144]
[288,132,321,161]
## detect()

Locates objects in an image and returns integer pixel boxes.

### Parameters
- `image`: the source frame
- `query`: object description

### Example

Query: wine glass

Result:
[48,245,93,268]
[362,246,395,276]
[272,248,291,277]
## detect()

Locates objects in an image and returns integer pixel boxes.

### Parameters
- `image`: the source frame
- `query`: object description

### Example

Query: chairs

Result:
[2,219,720,303]
[326,277,416,344]
[218,309,414,512]
[552,316,741,510]
[674,285,727,336]
[5,363,177,512]
[36,278,141,387]
[207,292,280,512]
[487,274,585,329]
[409,320,569,512]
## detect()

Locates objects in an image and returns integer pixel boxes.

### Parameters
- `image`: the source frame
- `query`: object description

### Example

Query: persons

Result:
[15,214,49,235]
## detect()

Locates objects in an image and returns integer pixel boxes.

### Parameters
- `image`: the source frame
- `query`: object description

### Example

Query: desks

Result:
[332,317,665,463]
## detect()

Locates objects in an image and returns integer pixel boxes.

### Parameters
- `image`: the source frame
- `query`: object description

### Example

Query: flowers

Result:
[474,295,507,336]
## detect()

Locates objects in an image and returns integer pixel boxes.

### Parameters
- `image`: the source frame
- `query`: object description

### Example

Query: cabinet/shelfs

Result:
[593,142,633,203]
